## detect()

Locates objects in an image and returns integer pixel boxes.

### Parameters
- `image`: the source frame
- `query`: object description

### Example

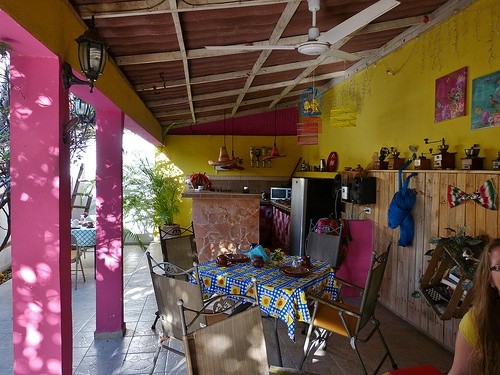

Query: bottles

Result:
[80,214,85,221]
[320,159,325,171]
[301,159,305,171]
[249,146,272,167]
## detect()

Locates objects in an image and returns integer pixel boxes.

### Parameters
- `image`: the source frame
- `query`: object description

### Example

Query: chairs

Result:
[146,250,253,375]
[177,277,318,375]
[71,234,86,290]
[299,241,399,375]
[301,219,342,335]
[150,221,200,328]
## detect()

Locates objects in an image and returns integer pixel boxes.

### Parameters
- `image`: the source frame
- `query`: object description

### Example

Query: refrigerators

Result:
[287,177,337,257]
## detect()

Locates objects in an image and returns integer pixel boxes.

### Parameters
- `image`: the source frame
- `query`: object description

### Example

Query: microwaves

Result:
[270,188,291,200]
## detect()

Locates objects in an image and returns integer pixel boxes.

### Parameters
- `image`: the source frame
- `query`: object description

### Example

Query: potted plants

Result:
[122,157,189,239]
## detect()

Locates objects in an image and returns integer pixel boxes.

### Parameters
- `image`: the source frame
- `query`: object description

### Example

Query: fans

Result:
[203,0,402,61]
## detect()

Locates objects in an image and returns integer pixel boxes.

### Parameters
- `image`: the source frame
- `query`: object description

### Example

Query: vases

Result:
[190,180,203,189]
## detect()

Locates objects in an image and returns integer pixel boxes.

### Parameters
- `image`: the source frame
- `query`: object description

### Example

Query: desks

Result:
[71,221,96,280]
[189,245,343,368]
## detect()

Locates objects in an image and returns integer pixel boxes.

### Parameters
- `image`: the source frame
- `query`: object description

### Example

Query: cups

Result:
[72,219,79,226]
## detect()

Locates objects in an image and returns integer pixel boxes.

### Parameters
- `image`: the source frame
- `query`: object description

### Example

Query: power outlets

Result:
[365,206,372,215]
[341,186,348,199]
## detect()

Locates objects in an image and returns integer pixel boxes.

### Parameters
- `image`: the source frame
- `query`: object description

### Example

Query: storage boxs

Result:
[418,235,482,321]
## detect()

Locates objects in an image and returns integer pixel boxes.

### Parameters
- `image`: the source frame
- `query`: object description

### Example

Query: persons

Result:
[447,237,500,375]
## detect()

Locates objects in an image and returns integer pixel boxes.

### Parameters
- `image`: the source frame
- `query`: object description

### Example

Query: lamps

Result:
[62,15,109,94]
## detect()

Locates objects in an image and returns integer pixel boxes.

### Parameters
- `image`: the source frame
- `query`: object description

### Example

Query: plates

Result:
[327,152,338,171]
[280,266,310,276]
[225,253,248,262]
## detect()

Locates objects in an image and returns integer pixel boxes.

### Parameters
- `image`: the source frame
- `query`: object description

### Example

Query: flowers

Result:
[190,171,213,189]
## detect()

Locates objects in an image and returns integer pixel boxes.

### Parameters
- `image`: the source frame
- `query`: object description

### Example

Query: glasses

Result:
[489,264,500,272]
